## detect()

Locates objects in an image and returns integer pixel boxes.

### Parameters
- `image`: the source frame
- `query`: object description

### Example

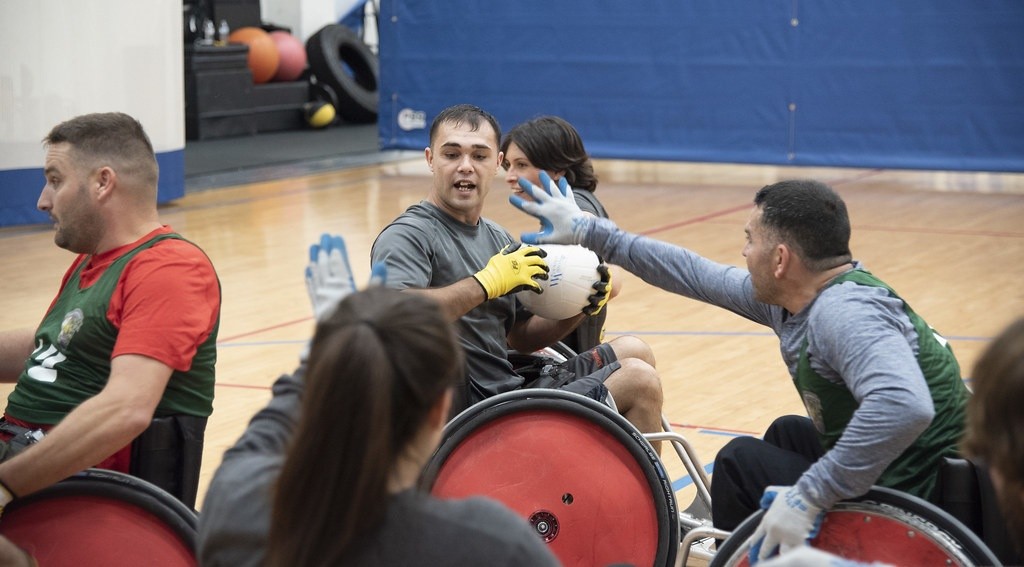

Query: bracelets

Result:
[0,479,17,500]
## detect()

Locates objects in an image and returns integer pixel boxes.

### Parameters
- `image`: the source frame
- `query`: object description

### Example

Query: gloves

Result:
[509,170,591,246]
[583,255,613,317]
[305,233,387,323]
[472,242,550,303]
[748,485,826,567]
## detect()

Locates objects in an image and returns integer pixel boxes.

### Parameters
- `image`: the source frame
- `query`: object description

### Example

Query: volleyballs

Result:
[514,232,602,321]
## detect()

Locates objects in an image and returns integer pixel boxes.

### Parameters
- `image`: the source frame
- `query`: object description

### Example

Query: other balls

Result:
[303,100,336,128]
[227,26,279,84]
[268,30,307,82]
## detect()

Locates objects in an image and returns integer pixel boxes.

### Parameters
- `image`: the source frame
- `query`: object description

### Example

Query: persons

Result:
[370,106,663,456]
[508,171,979,567]
[0,112,222,508]
[498,117,621,352]
[966,317,1024,567]
[194,232,558,567]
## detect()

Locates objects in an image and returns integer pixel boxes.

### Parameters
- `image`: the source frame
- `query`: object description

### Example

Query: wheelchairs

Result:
[0,419,206,567]
[675,386,1003,567]
[411,336,721,567]
